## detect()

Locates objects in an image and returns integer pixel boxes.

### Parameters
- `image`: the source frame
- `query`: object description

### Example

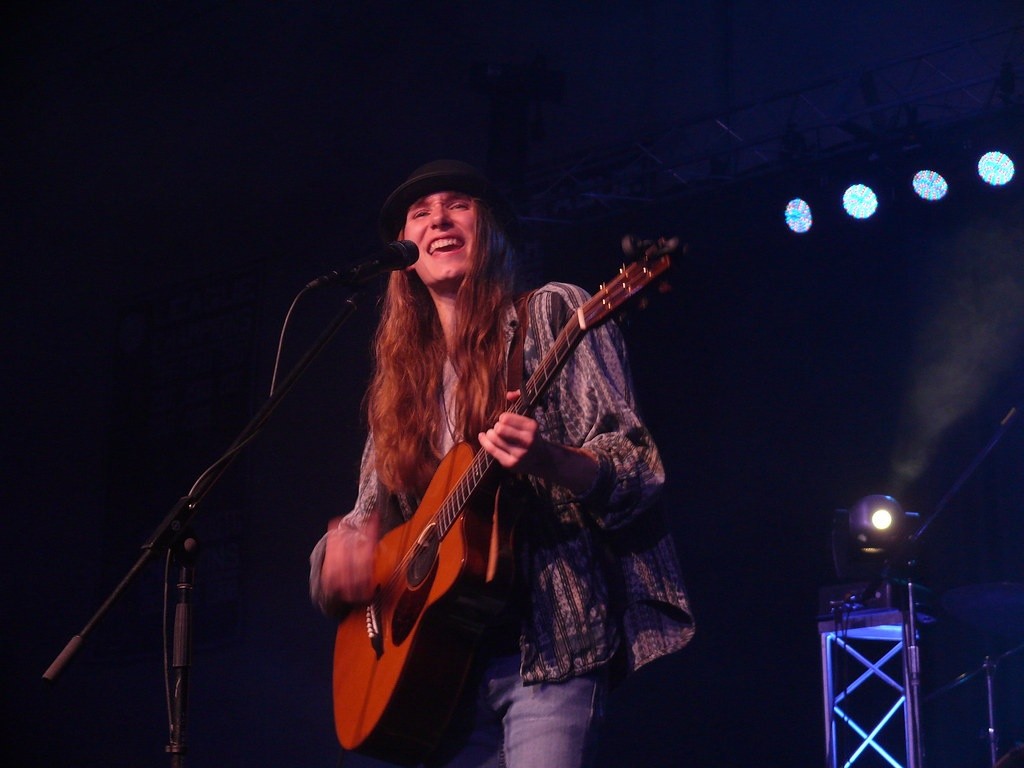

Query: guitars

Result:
[323,230,688,768]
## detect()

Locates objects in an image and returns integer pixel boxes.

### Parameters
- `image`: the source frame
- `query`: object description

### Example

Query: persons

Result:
[303,159,695,767]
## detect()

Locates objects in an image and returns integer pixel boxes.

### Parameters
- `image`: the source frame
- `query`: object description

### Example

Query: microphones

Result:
[309,240,419,288]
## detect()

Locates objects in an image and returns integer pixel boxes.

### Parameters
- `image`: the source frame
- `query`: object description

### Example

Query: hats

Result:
[377,158,522,252]
